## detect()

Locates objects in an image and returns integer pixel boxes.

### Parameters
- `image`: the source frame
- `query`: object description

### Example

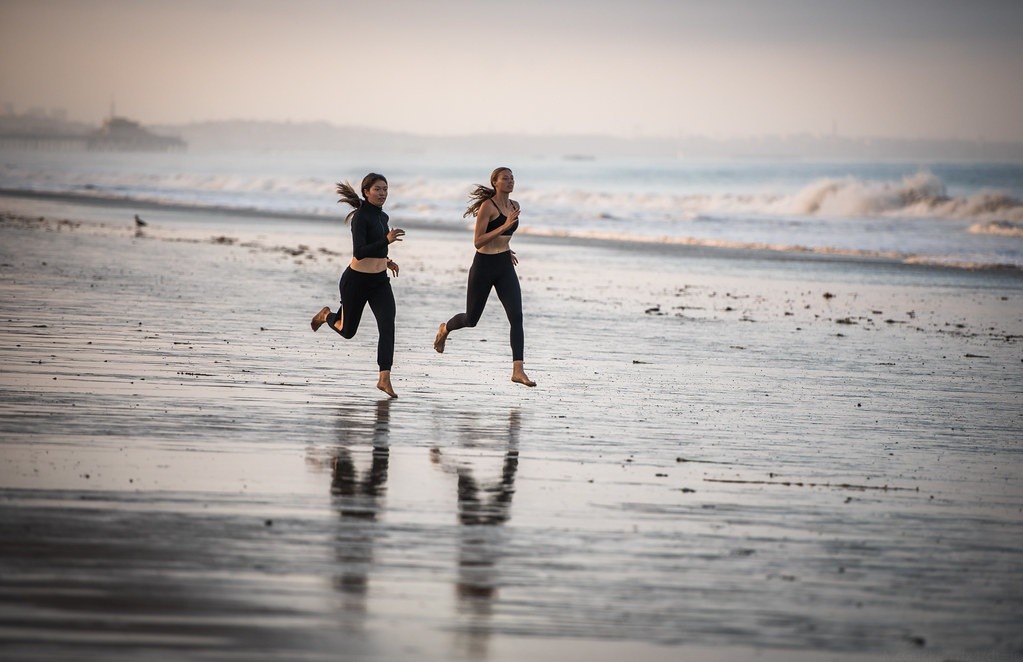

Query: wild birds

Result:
[134,214,148,231]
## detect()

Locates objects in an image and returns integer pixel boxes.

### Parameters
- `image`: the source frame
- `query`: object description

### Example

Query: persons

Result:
[311,173,406,398]
[433,167,537,388]
